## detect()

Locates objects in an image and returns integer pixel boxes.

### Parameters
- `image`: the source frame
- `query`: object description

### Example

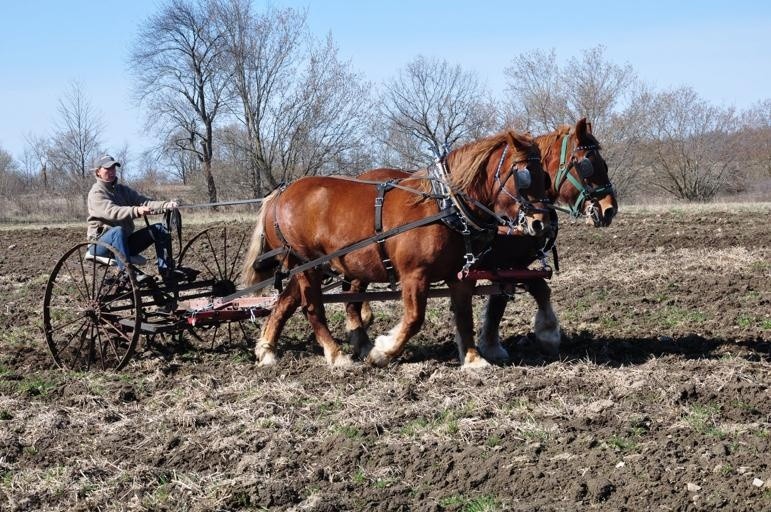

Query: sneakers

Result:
[123,265,154,282]
[163,267,192,281]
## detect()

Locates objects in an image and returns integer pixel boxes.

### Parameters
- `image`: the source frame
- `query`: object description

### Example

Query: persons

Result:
[85,151,192,284]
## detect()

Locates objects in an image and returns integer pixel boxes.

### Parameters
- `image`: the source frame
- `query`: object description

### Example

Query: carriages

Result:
[40,118,620,376]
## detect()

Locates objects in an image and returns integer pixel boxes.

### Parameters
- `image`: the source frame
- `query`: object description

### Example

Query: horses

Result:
[342,116,619,367]
[240,130,552,370]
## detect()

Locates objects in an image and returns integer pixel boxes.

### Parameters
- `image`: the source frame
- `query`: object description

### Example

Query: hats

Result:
[96,155,122,169]
